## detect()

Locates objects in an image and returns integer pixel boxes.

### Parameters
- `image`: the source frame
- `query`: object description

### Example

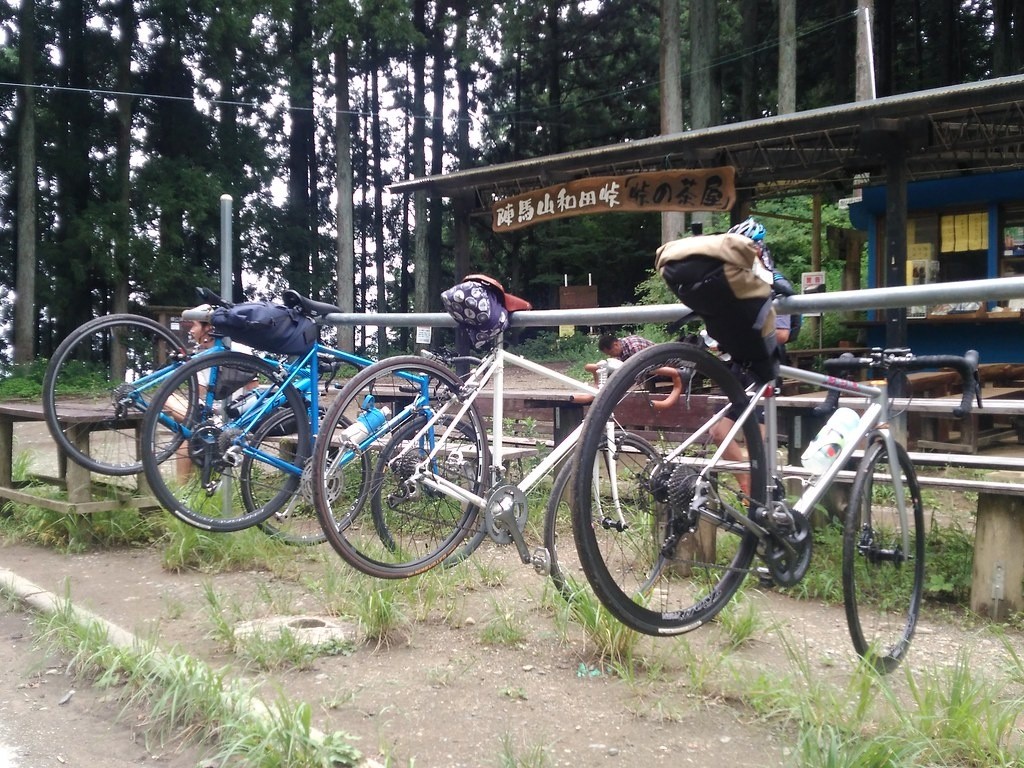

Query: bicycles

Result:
[568,240,986,677]
[311,273,674,622]
[139,288,501,571]
[40,286,375,549]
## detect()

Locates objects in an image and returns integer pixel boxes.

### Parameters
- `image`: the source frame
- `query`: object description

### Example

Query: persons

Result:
[600,335,681,392]
[156,321,259,492]
[699,220,789,512]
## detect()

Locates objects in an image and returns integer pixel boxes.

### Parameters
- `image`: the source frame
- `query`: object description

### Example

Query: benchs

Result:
[792,369,961,457]
[356,384,599,510]
[0,395,174,549]
[918,385,1024,458]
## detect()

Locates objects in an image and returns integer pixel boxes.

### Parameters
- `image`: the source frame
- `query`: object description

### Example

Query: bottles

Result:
[226,391,260,418]
[342,407,391,448]
[803,406,861,480]
